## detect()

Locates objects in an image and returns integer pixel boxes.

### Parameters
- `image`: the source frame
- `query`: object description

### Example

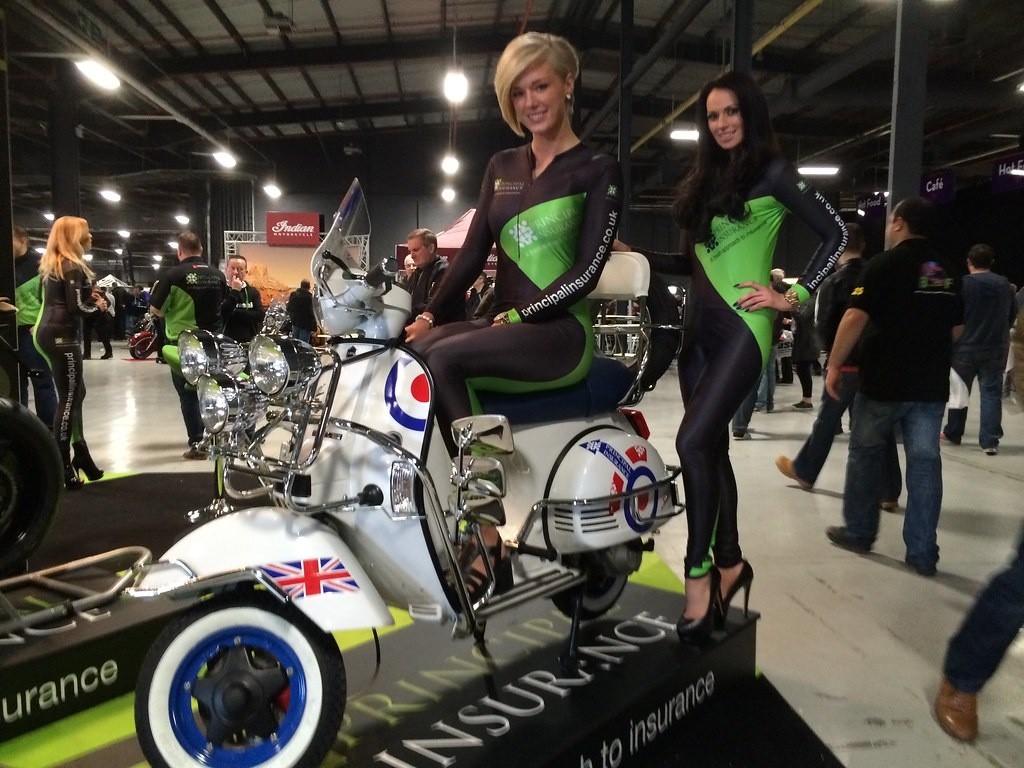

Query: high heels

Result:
[59,448,85,489]
[676,563,727,638]
[448,531,515,603]
[712,559,755,624]
[71,440,105,480]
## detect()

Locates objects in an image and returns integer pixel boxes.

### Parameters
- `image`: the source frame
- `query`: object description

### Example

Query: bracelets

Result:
[415,314,433,328]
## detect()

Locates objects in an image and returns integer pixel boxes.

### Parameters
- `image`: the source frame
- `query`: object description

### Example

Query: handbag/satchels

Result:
[946,366,969,411]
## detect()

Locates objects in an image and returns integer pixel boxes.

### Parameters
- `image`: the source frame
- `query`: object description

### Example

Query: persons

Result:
[776,223,902,509]
[940,244,1018,453]
[732,269,821,440]
[287,279,317,344]
[149,231,229,460]
[101,282,149,340]
[80,315,113,360]
[405,28,625,602]
[933,519,1024,739]
[221,254,265,444]
[401,228,466,322]
[466,272,490,311]
[13,225,58,433]
[825,197,965,576]
[33,216,107,486]
[612,73,849,639]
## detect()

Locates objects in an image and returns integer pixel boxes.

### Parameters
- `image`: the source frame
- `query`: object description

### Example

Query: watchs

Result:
[784,290,799,309]
[494,312,510,325]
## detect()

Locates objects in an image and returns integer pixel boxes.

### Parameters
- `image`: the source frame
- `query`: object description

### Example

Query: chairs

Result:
[476,246,651,423]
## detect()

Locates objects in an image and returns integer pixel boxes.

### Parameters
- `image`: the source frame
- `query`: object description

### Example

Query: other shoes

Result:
[985,447,997,456]
[101,351,113,359]
[880,499,899,510]
[184,446,207,460]
[776,455,814,492]
[733,425,750,441]
[779,377,793,384]
[827,522,870,555]
[939,429,950,441]
[793,401,815,412]
[905,555,938,576]
[936,669,979,743]
[81,351,91,360]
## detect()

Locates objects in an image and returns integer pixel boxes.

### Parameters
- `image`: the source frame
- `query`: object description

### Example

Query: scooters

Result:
[126,179,689,768]
